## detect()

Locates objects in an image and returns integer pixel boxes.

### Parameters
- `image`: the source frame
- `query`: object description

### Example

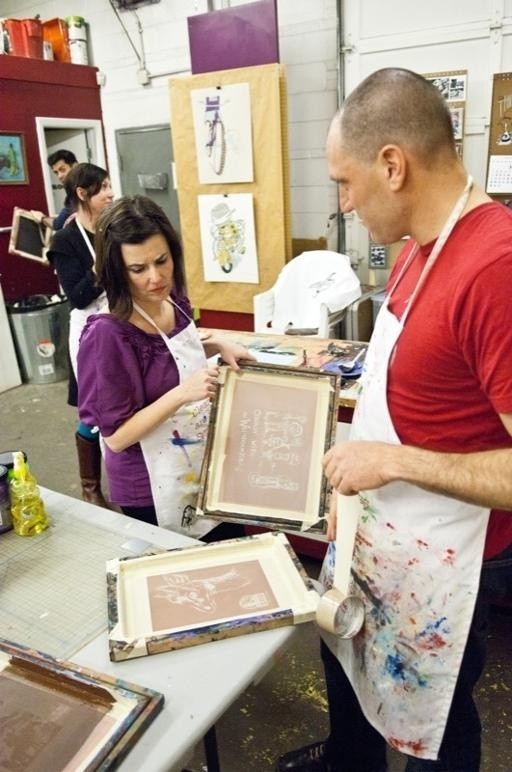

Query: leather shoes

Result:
[274,738,335,772]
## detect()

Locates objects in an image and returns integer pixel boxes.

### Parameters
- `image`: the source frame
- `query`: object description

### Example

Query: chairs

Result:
[253,249,363,339]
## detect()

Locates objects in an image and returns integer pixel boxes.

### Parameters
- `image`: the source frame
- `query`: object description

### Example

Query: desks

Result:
[0,485,311,772]
[196,326,370,561]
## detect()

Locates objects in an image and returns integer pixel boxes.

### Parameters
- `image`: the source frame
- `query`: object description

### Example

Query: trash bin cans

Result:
[8,292,71,385]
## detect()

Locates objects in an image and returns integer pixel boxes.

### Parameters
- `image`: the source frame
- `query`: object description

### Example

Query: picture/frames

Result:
[0,130,30,185]
[195,358,342,534]
[368,243,387,269]
[9,206,52,265]
[0,639,165,772]
[105,532,321,662]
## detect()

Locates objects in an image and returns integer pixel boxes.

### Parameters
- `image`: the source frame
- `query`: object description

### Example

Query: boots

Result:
[76,432,108,511]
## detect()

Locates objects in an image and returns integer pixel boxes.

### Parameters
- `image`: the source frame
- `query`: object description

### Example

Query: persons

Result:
[7,142,22,176]
[78,193,246,544]
[31,150,81,230]
[44,162,115,510]
[276,69,512,771]
